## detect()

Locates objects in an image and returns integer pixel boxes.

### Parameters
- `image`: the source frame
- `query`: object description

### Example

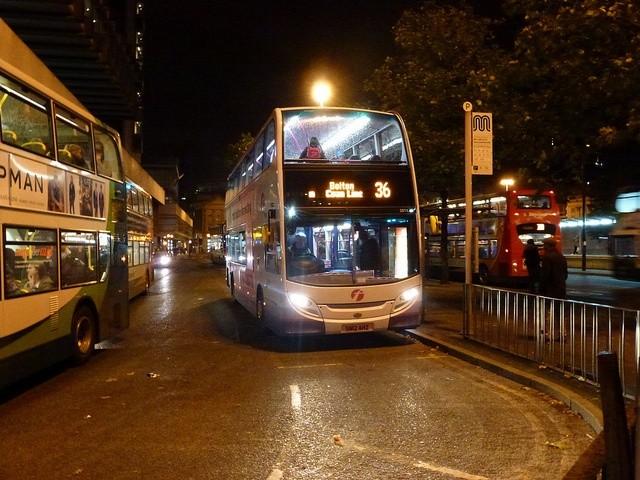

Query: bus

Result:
[0,13,130,374]
[207,223,227,265]
[124,176,154,301]
[224,105,424,340]
[420,189,562,286]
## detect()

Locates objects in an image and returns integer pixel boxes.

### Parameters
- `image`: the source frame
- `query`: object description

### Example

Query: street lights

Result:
[500,178,514,191]
[575,141,603,270]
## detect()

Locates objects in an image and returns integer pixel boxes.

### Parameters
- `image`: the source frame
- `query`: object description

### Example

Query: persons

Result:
[5,264,21,296]
[93,183,98,217]
[298,137,326,163]
[25,263,54,290]
[69,175,75,214]
[492,241,497,256]
[99,184,104,218]
[51,175,64,212]
[95,142,111,176]
[354,229,378,270]
[64,144,89,170]
[83,185,91,212]
[537,238,569,338]
[286,232,312,259]
[522,239,540,294]
